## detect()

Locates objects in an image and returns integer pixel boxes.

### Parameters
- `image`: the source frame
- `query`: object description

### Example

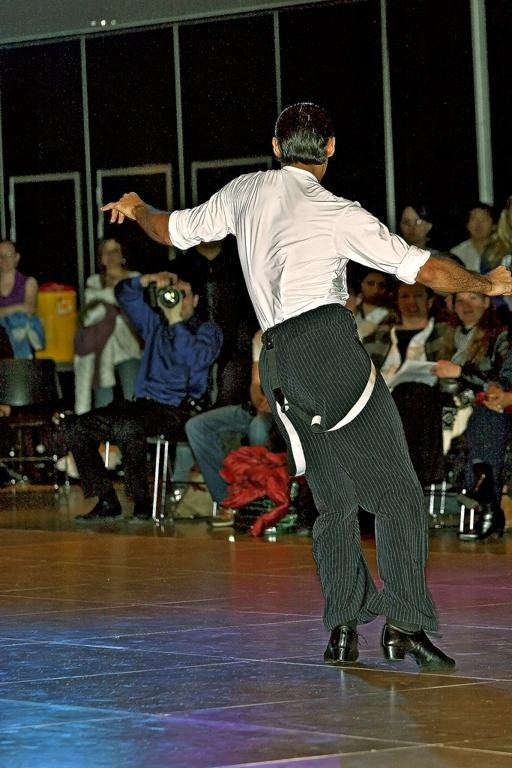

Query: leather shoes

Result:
[73,499,123,523]
[324,625,359,664]
[128,500,152,524]
[381,623,456,669]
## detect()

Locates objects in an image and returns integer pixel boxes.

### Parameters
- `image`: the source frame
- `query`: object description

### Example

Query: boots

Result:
[457,504,506,542]
[463,462,497,501]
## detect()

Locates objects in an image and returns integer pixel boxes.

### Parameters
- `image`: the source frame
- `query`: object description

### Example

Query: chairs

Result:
[1,356,76,507]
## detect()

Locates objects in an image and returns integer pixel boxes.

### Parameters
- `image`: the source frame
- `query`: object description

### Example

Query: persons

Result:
[93,99,512,671]
[69,236,292,533]
[0,241,36,360]
[345,197,512,536]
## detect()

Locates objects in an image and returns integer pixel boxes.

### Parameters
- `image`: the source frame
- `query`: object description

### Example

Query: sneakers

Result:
[208,510,234,527]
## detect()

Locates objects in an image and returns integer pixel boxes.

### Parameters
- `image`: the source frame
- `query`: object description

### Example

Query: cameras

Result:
[149,279,186,308]
